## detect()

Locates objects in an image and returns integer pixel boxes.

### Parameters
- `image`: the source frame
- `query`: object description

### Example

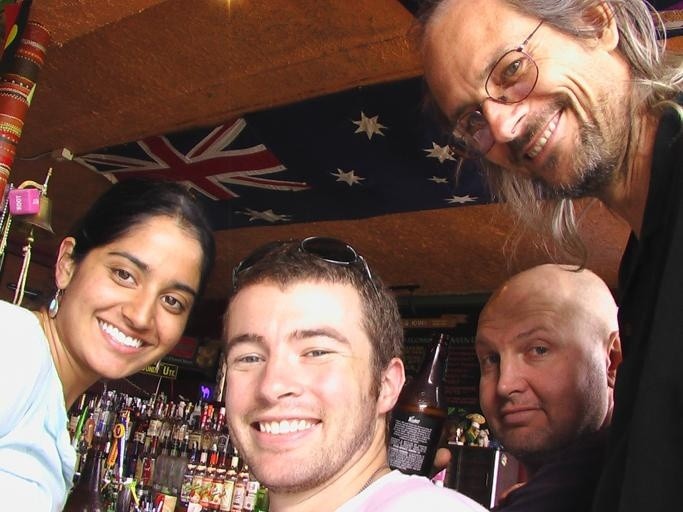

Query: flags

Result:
[72,70,509,229]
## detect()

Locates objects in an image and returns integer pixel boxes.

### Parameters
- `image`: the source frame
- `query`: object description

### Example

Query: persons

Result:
[0,173,216,512]
[224,237,480,512]
[473,264,621,511]
[394,0,683,509]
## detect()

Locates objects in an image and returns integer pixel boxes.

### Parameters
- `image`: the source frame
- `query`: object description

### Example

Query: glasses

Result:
[444,21,544,158]
[232,235,383,325]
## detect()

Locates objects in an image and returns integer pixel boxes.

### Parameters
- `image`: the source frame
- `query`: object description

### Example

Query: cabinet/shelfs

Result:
[67,354,271,512]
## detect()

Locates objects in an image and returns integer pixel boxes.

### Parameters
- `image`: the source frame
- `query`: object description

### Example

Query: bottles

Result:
[199,450,217,512]
[231,461,249,512]
[94,400,111,437]
[179,440,199,507]
[63,447,105,512]
[219,453,239,512]
[152,439,170,492]
[70,393,86,433]
[133,399,226,451]
[94,401,101,422]
[84,413,94,444]
[125,432,138,478]
[189,448,207,506]
[79,436,87,454]
[241,468,260,512]
[88,400,94,413]
[133,434,152,485]
[104,411,128,482]
[386,331,450,476]
[207,451,227,512]
[163,439,178,494]
[175,439,188,498]
[139,436,158,488]
[254,484,269,512]
